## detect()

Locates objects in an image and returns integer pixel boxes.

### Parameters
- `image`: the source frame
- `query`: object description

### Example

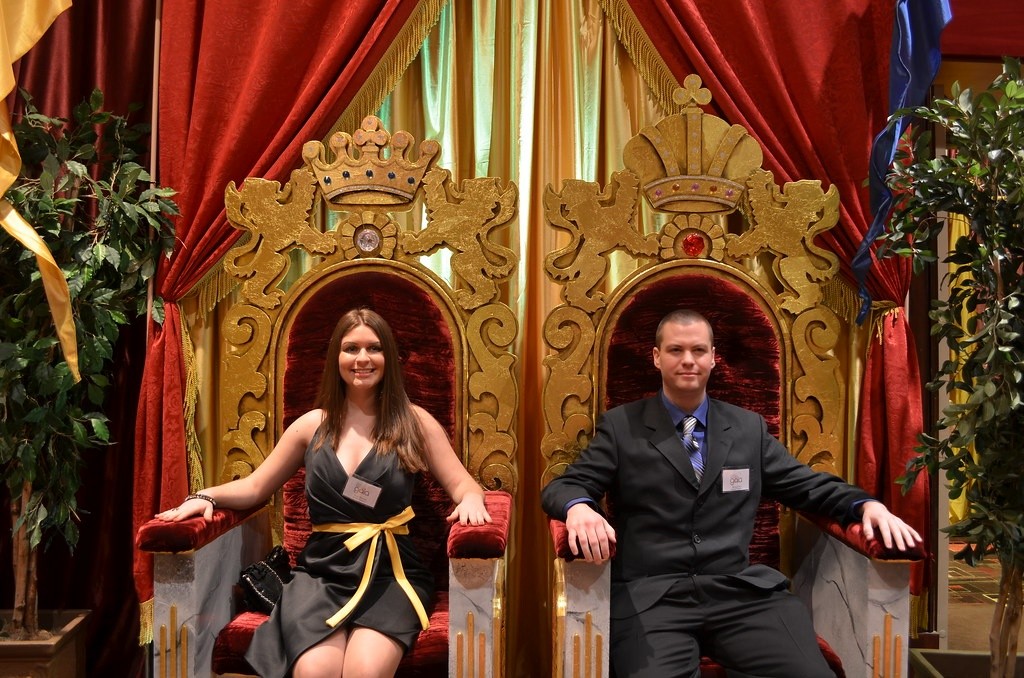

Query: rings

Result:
[171,507,180,511]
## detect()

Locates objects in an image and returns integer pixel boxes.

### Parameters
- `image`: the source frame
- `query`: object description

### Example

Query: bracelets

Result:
[185,494,217,508]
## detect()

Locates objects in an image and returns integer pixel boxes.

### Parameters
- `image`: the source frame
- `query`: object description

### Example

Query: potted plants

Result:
[0,85,185,675]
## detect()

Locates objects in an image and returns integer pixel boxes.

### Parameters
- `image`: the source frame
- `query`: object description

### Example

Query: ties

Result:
[682,416,704,486]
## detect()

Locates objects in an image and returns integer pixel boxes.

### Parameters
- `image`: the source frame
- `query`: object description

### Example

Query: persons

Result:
[154,309,493,677]
[541,308,926,678]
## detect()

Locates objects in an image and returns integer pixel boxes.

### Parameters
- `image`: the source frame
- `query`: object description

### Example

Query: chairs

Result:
[134,113,520,675]
[540,74,929,678]
